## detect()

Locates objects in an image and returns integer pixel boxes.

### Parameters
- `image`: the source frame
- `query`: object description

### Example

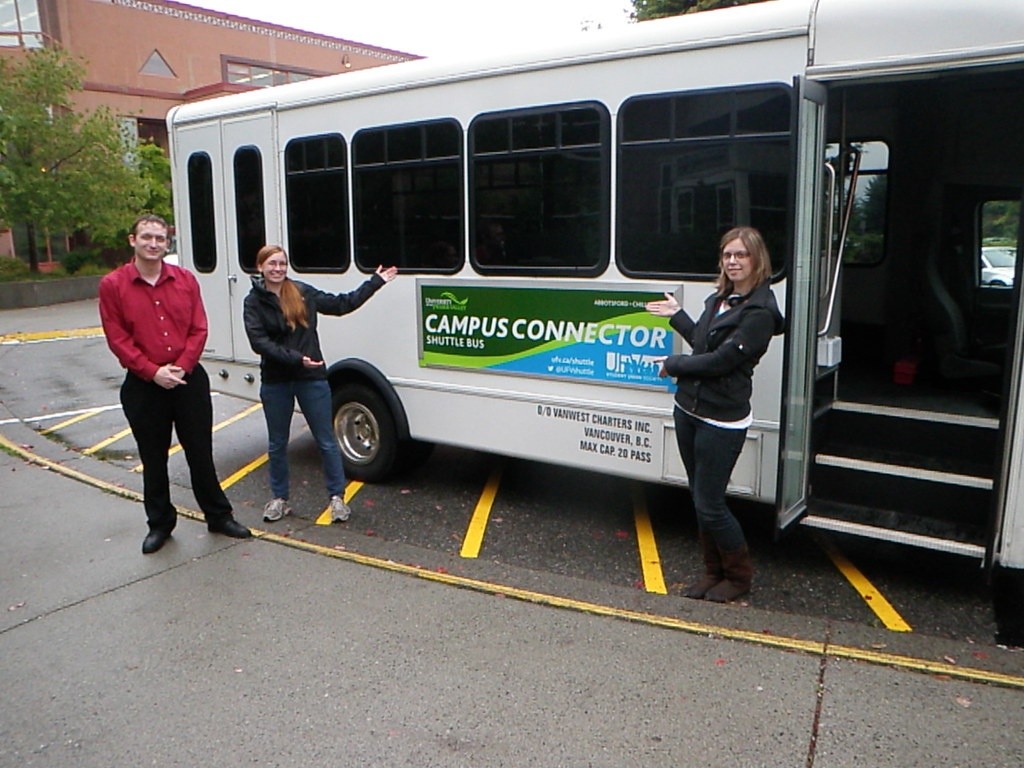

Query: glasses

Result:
[722,251,748,260]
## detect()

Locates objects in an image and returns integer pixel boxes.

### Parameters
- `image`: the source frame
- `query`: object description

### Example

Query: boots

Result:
[682,529,721,601]
[707,546,752,602]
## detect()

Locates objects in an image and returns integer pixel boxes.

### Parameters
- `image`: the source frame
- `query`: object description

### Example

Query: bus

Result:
[167,0,1024,570]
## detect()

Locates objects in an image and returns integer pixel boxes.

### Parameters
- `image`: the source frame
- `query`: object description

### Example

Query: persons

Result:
[243,245,398,522]
[644,226,785,601]
[98,215,252,554]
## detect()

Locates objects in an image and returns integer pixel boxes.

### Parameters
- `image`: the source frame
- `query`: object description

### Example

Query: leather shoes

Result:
[207,520,250,538]
[143,525,167,553]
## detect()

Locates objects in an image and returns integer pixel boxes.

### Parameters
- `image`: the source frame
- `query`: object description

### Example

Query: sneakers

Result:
[330,495,350,523]
[263,497,291,521]
